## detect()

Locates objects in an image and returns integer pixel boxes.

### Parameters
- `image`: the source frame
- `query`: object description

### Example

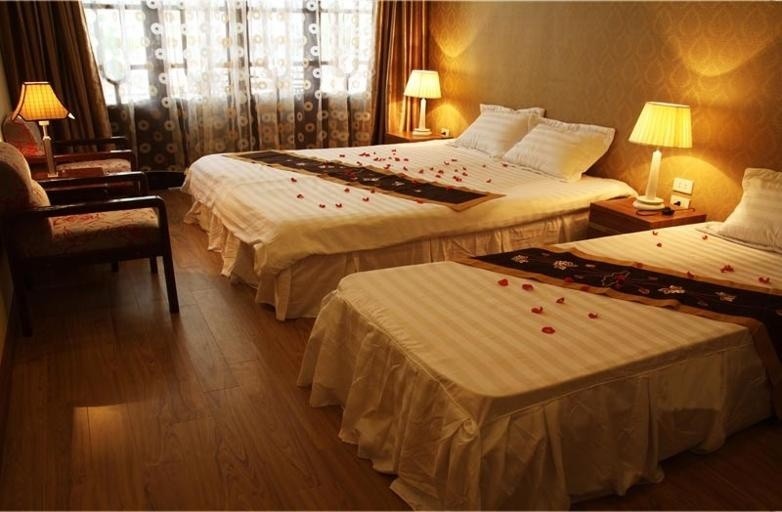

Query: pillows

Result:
[498,115,619,185]
[692,167,782,255]
[444,101,544,160]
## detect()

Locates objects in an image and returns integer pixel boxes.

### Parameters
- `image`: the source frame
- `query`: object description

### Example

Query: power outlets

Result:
[672,177,693,195]
[668,195,690,209]
[439,128,449,137]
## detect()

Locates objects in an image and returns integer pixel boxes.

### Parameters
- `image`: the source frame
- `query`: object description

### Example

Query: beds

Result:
[178,100,641,324]
[294,219,782,512]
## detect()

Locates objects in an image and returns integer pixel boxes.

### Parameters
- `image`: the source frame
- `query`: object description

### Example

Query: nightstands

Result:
[383,130,453,144]
[585,196,708,239]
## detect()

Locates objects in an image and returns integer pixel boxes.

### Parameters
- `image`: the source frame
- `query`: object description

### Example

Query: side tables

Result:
[31,166,120,282]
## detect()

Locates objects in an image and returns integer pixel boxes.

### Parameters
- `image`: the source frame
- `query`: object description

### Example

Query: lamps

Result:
[402,69,444,137]
[11,81,74,180]
[625,99,693,212]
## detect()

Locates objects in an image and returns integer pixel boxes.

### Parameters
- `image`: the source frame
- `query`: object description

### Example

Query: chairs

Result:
[0,109,140,188]
[0,140,180,334]
